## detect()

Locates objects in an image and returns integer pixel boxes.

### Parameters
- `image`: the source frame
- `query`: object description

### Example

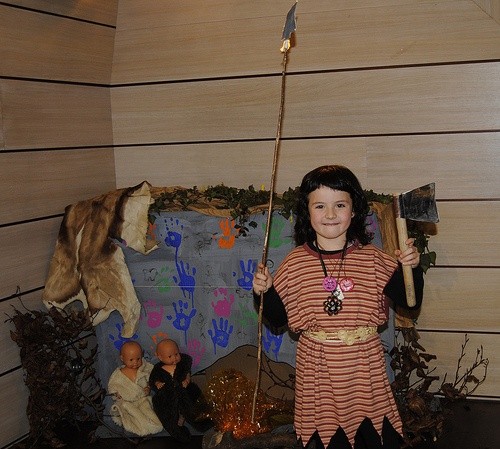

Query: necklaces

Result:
[322,276,354,316]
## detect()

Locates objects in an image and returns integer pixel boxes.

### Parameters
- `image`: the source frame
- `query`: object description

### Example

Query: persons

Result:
[108,341,164,436]
[149,339,215,444]
[252,166,424,449]
